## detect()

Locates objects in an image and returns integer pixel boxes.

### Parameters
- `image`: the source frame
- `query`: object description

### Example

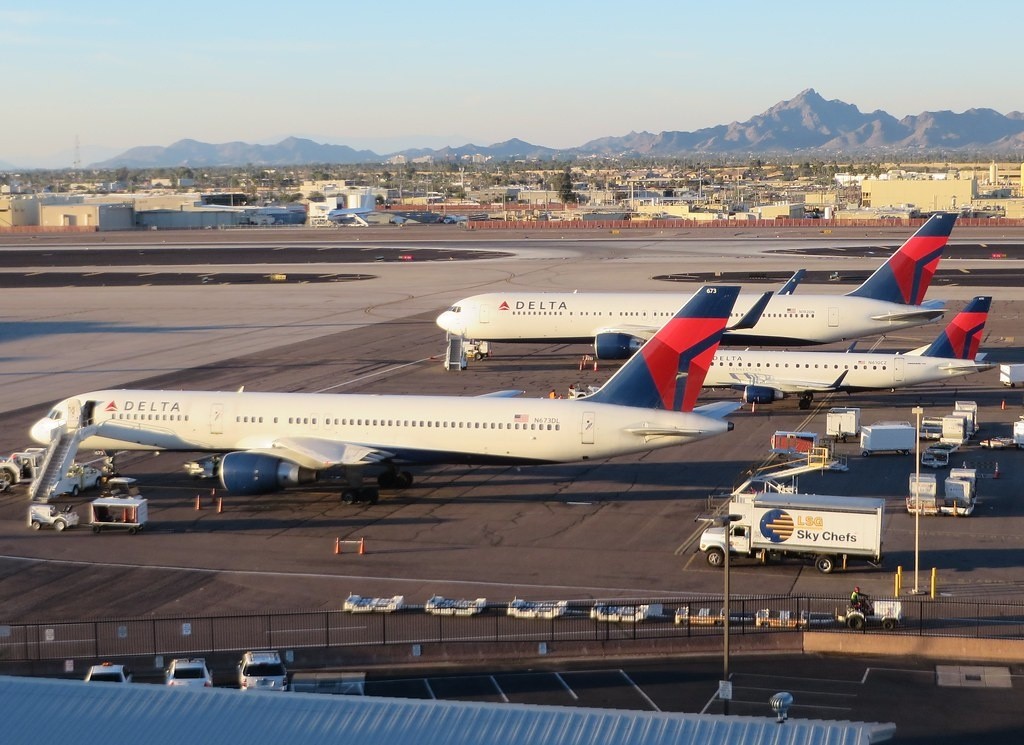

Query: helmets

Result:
[854,587,860,591]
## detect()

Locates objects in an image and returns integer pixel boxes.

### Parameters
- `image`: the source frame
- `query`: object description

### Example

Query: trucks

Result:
[697,490,887,574]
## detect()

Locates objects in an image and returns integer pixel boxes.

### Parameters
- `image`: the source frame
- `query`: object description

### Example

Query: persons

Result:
[550,389,556,399]
[558,394,563,400]
[851,587,869,609]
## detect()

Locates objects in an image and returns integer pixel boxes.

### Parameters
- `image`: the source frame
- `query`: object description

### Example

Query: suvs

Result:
[165,656,214,689]
[238,648,289,691]
[82,661,132,684]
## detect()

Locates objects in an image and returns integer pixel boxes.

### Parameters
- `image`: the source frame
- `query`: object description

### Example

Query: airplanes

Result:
[28,279,746,499]
[700,296,996,403]
[436,210,961,367]
